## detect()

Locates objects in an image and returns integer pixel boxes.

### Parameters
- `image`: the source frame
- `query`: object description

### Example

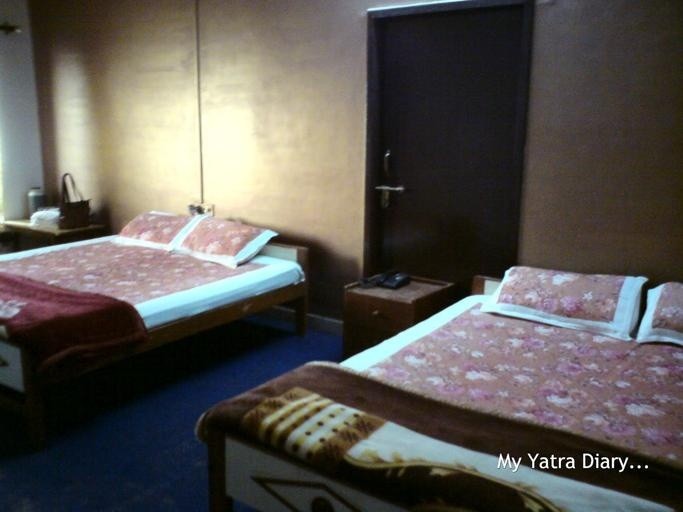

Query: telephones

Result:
[376,270,410,289]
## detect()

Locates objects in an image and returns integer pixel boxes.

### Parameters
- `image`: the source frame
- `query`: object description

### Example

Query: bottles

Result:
[27,186,45,220]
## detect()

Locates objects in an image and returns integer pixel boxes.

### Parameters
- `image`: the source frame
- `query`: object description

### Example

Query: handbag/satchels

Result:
[59,173,91,229]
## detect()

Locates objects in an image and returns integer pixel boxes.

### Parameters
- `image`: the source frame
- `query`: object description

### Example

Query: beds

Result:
[198,272,682,512]
[0,234,309,460]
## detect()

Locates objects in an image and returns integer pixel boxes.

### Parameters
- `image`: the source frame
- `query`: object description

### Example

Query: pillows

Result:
[176,214,279,267]
[481,265,648,342]
[633,283,683,346]
[119,210,201,252]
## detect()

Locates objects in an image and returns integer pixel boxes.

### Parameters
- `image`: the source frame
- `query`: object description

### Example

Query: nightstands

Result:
[343,271,453,359]
[1,218,107,252]
